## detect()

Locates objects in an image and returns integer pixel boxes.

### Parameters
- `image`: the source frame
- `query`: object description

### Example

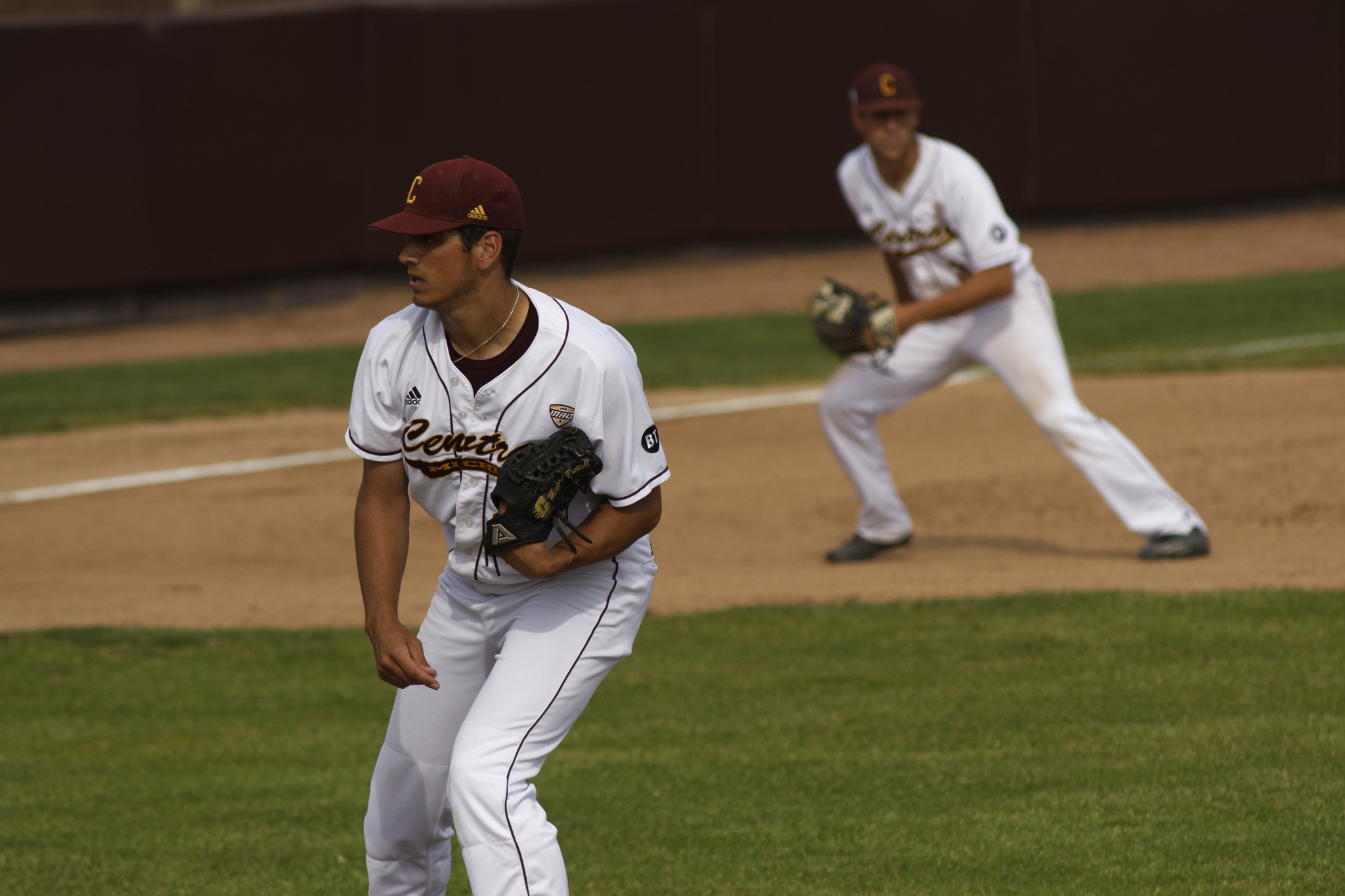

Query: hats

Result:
[851,66,921,116]
[369,153,526,236]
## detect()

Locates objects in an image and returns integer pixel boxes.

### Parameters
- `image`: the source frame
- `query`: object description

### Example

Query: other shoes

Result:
[1139,526,1210,557]
[830,532,911,562]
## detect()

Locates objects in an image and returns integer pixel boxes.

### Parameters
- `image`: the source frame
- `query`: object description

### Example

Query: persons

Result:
[815,62,1211,561]
[342,155,671,896]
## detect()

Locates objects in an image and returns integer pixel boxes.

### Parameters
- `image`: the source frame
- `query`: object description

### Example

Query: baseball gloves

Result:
[811,275,900,354]
[480,429,600,553]
